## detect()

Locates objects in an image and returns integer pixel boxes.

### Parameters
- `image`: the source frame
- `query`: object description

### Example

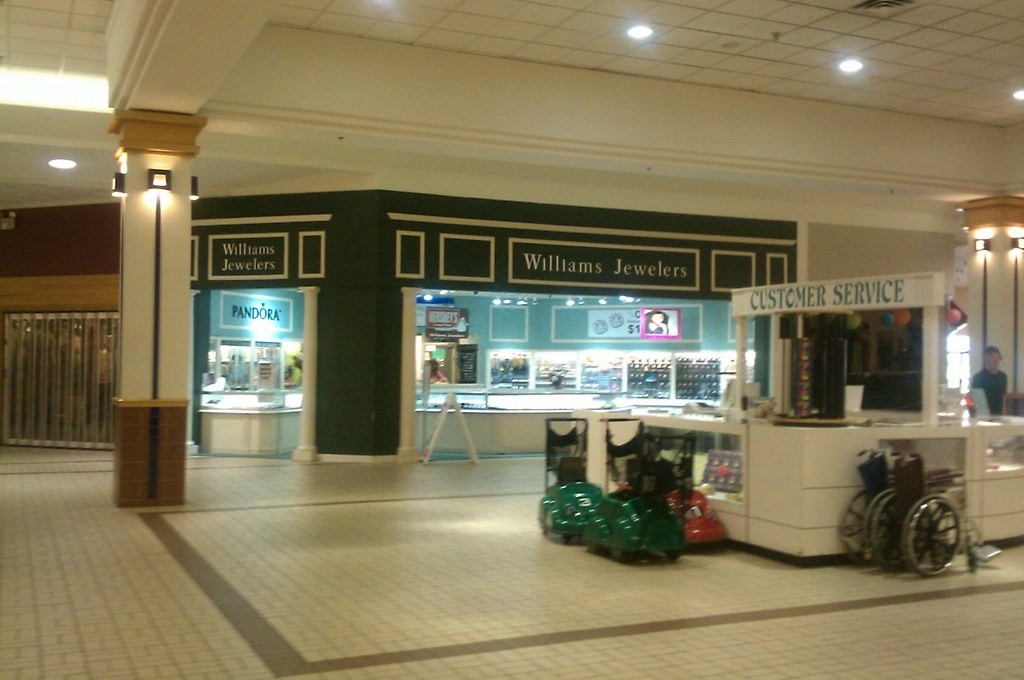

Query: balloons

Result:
[893,309,912,325]
[881,313,895,325]
[845,311,862,329]
[948,308,962,325]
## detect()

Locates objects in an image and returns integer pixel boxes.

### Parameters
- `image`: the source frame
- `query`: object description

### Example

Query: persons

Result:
[420,358,448,384]
[645,311,668,335]
[972,346,1008,416]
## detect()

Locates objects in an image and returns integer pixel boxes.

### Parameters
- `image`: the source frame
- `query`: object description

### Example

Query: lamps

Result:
[111,172,125,197]
[148,169,171,191]
[191,176,199,201]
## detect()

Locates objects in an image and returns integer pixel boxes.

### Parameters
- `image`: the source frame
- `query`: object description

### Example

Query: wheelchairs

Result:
[840,447,1003,577]
